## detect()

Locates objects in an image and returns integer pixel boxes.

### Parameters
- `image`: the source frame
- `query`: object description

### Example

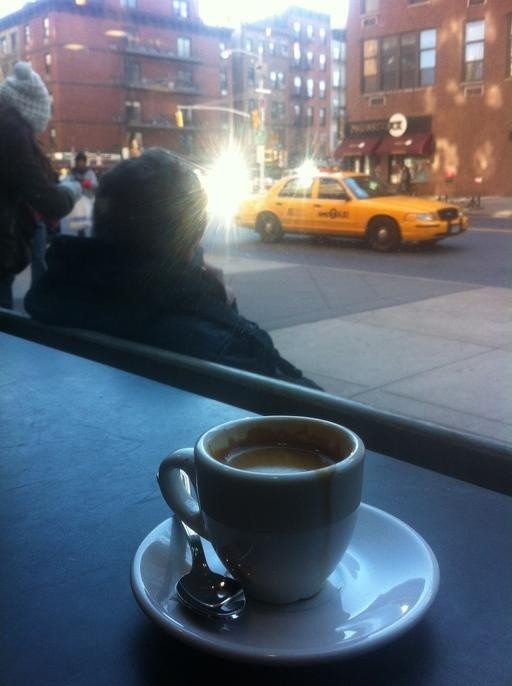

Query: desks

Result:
[0,307,511,685]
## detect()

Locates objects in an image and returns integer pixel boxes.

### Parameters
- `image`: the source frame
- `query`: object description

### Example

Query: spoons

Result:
[175,468,246,621]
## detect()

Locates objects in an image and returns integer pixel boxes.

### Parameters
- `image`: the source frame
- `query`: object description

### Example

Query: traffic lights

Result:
[249,108,262,131]
[174,110,185,129]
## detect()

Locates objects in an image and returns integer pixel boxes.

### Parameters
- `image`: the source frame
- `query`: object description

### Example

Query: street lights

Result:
[222,48,267,196]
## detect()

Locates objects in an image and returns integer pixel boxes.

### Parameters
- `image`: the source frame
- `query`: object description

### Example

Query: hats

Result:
[86,143,210,261]
[0,58,54,135]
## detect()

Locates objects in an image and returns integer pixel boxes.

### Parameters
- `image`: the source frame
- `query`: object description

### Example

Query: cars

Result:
[234,171,469,253]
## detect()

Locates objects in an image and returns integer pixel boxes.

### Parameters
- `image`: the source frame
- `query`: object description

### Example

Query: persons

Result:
[20,143,332,400]
[55,149,99,240]
[394,155,412,196]
[0,59,86,318]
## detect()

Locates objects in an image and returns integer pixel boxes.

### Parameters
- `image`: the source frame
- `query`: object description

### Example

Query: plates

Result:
[130,501,440,664]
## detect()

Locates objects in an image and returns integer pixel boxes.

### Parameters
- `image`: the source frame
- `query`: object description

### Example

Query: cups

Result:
[158,415,366,606]
